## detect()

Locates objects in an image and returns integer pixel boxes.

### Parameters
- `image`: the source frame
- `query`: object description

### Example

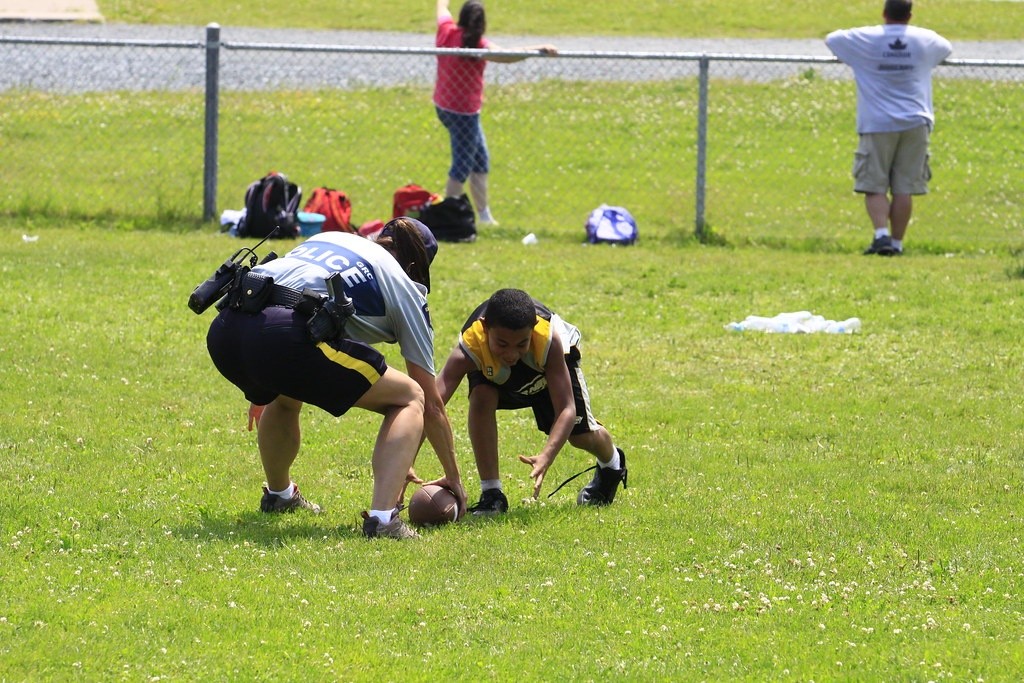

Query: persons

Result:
[826,0,953,256]
[433,0,559,224]
[207,216,468,541]
[397,289,627,516]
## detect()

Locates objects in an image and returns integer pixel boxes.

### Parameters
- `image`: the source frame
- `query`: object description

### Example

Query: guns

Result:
[214,250,279,313]
[306,270,356,342]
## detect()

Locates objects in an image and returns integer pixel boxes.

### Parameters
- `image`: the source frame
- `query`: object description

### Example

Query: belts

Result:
[264,286,302,308]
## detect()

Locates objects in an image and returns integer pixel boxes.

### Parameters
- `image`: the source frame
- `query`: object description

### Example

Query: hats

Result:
[381,217,439,294]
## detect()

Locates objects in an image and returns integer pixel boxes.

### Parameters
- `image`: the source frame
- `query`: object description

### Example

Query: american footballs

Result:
[410,484,459,525]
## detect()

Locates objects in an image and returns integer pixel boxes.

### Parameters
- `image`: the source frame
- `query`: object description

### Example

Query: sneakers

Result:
[360,502,423,540]
[261,483,327,517]
[547,447,628,506]
[469,488,508,516]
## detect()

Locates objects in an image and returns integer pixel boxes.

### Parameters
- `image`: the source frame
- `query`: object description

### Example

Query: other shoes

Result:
[863,237,903,254]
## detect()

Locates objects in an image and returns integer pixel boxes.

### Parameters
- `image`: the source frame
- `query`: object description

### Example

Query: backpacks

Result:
[393,184,436,222]
[586,204,639,245]
[237,172,303,239]
[305,188,351,233]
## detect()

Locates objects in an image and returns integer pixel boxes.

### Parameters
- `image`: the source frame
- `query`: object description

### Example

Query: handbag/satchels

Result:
[421,194,478,242]
